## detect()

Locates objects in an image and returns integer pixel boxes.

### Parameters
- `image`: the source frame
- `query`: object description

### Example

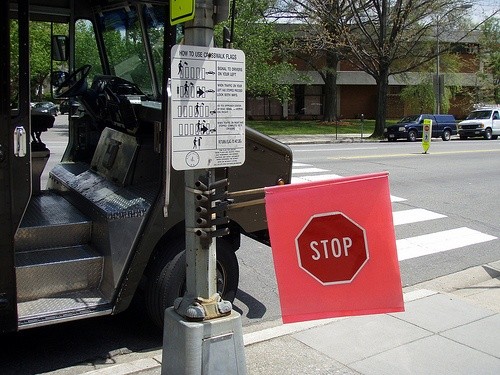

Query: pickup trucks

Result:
[384,114,457,141]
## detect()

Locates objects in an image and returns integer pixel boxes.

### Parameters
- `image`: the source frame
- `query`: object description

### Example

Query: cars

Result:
[30,102,59,116]
[60,99,82,115]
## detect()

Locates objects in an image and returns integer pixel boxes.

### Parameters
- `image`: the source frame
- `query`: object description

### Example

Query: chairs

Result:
[9,107,56,150]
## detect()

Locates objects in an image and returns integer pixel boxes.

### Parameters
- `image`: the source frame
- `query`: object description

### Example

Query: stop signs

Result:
[293,210,369,286]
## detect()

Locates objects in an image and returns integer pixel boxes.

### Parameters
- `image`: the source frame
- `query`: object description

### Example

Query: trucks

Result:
[0,1,293,349]
[457,104,500,140]
